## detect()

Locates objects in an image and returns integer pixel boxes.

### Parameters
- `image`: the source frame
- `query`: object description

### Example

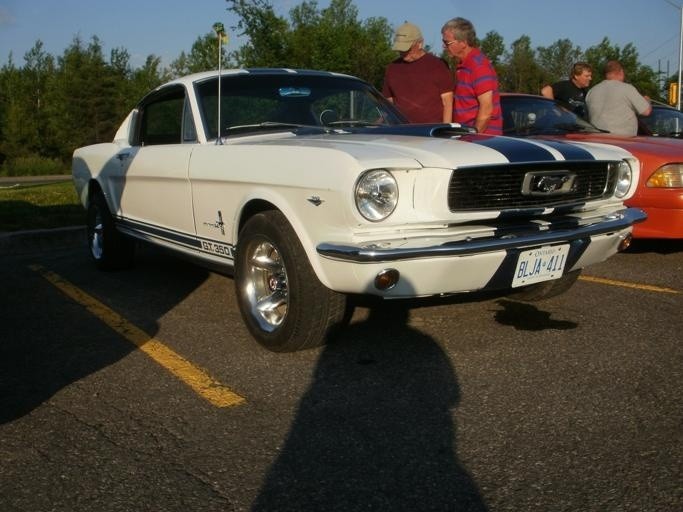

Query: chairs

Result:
[274,96,318,128]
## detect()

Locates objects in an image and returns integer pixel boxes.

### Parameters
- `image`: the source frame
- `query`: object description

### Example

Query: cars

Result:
[69,26,642,355]
[497,91,683,242]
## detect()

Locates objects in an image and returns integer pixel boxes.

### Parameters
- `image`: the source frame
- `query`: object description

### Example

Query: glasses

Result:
[442,38,460,47]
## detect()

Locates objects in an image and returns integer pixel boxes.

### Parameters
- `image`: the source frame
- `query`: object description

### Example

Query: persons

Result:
[370,22,455,128]
[540,62,596,124]
[584,60,652,137]
[440,16,502,136]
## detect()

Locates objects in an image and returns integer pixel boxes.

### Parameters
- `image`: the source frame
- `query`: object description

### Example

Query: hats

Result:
[392,23,424,52]
